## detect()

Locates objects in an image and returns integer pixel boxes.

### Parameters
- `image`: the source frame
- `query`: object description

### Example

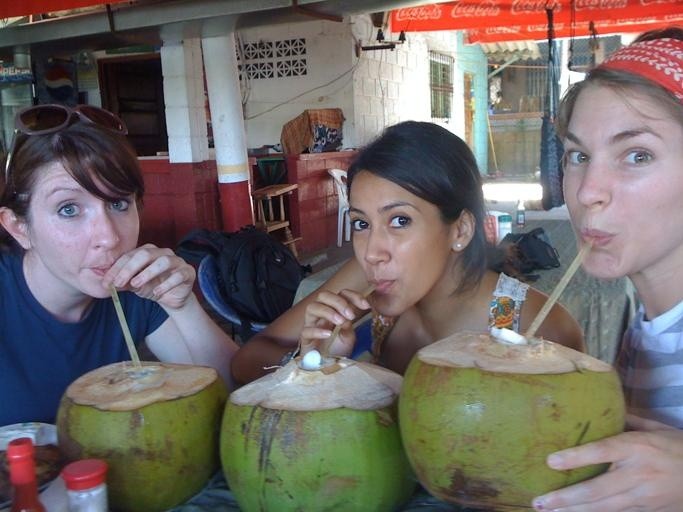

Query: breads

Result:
[0,443,62,500]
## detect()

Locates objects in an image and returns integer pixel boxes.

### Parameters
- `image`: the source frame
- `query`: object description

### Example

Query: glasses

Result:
[4,104,129,192]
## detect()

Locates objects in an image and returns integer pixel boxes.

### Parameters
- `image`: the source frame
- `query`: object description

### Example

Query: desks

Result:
[300,220,628,366]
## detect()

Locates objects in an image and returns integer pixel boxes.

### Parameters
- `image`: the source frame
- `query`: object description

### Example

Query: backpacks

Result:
[175,223,306,325]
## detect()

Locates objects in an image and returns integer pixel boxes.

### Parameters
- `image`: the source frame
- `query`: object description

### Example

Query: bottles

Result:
[515,199,526,228]
[60,457,110,512]
[7,436,50,512]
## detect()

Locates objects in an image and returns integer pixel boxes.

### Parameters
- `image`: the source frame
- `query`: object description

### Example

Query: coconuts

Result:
[396,325,629,511]
[55,359,229,509]
[219,344,418,511]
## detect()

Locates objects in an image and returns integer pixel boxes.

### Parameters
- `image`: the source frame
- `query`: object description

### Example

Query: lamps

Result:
[362,29,406,51]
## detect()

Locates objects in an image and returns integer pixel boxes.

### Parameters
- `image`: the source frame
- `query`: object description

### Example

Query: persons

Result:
[228,119,588,390]
[530,26,683,512]
[0,103,239,432]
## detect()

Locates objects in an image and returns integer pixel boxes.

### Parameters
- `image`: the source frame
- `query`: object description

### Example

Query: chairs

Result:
[197,254,326,334]
[327,168,350,248]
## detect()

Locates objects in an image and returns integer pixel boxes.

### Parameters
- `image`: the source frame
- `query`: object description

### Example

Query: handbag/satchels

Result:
[503,228,561,274]
